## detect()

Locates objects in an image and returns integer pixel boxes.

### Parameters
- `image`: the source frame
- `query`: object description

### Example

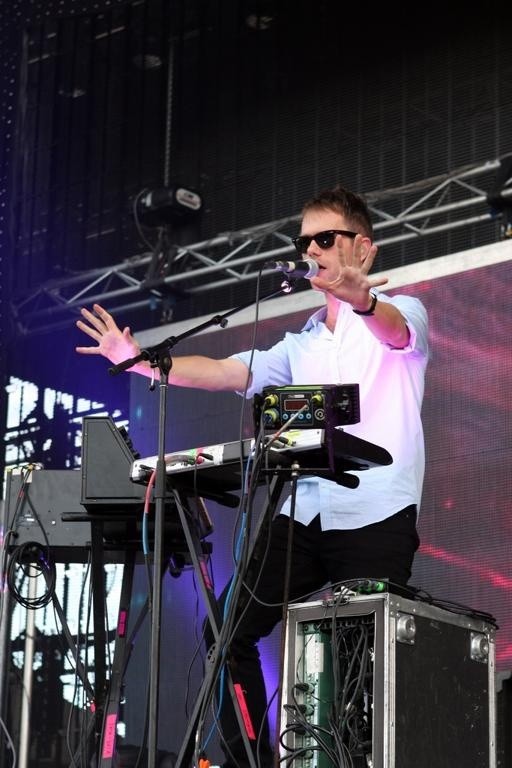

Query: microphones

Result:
[264,258,320,279]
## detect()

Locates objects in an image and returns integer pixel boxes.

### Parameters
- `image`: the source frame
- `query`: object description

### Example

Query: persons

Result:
[74,186,430,767]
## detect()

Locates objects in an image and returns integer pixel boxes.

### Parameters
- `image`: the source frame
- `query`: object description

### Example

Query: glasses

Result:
[291,229,358,255]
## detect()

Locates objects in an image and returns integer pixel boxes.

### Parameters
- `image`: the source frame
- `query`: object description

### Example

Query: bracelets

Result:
[353,293,378,316]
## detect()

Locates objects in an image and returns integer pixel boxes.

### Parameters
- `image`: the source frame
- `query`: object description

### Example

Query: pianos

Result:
[128,426,393,496]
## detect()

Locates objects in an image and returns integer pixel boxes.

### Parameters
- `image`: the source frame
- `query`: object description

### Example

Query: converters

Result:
[360,578,420,601]
